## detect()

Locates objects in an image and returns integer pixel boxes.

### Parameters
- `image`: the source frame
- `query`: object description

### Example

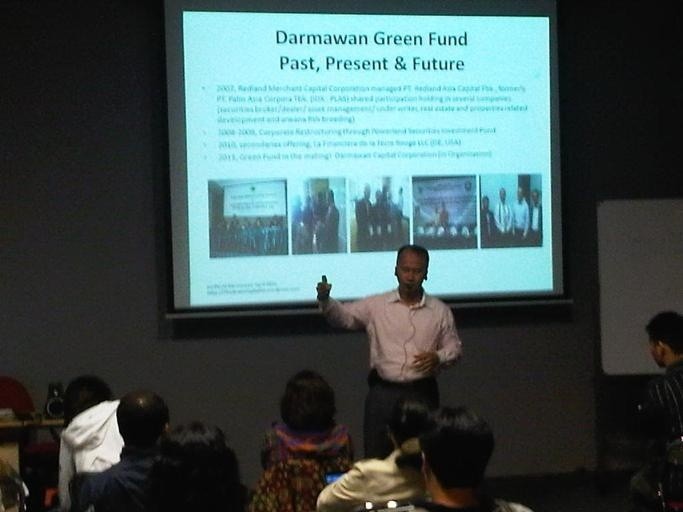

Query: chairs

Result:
[1,376,56,478]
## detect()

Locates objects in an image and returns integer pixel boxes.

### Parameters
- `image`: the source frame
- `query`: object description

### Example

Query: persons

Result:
[68,388,171,511]
[316,245,463,460]
[60,376,126,511]
[395,404,531,510]
[211,215,284,255]
[439,202,449,228]
[480,187,542,246]
[247,377,354,511]
[317,393,439,510]
[638,311,683,432]
[354,184,404,251]
[139,421,257,510]
[292,190,339,252]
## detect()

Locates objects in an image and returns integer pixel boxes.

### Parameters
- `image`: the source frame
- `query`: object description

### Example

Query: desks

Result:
[0,415,68,511]
